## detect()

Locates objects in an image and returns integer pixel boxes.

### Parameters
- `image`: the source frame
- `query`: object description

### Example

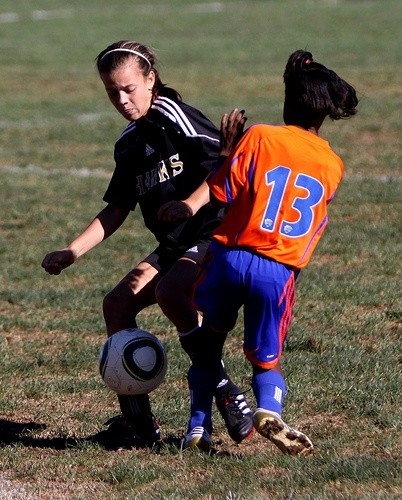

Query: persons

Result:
[42,41,255,452]
[183,49,359,455]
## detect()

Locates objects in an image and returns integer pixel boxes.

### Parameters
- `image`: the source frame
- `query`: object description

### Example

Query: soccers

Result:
[99,327,168,393]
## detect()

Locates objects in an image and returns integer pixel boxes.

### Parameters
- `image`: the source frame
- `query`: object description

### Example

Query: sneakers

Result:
[217,387,256,444]
[91,414,160,447]
[180,426,213,452]
[251,409,314,459]
